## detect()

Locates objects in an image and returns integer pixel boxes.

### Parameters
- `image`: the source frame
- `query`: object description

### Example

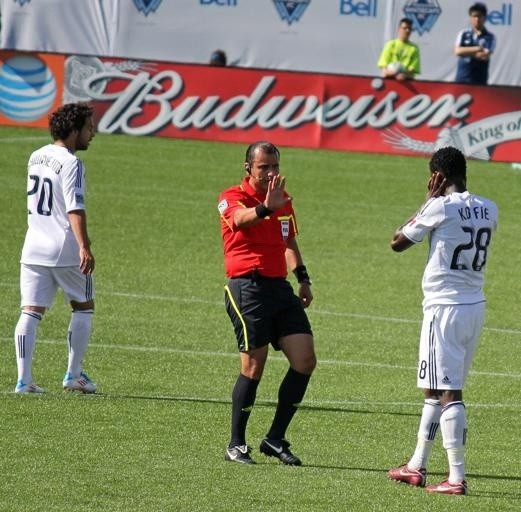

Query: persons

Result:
[389,146,499,494]
[13,101,97,394]
[454,2,496,86]
[216,140,317,465]
[377,18,421,81]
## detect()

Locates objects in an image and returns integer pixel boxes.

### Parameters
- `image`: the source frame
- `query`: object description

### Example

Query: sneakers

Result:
[15,383,45,393]
[224,444,256,464]
[259,438,302,466]
[387,463,427,487]
[63,372,97,393]
[424,480,467,495]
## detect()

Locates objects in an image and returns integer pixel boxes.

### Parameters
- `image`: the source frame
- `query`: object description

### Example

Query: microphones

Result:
[250,174,262,181]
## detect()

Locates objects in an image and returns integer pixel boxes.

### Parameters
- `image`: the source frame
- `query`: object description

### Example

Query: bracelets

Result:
[256,204,274,219]
[292,265,312,285]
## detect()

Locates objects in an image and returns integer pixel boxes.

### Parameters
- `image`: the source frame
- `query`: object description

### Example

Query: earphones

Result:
[247,167,251,173]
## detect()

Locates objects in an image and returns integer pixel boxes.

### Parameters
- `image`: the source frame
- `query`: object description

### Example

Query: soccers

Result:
[389,62,405,72]
